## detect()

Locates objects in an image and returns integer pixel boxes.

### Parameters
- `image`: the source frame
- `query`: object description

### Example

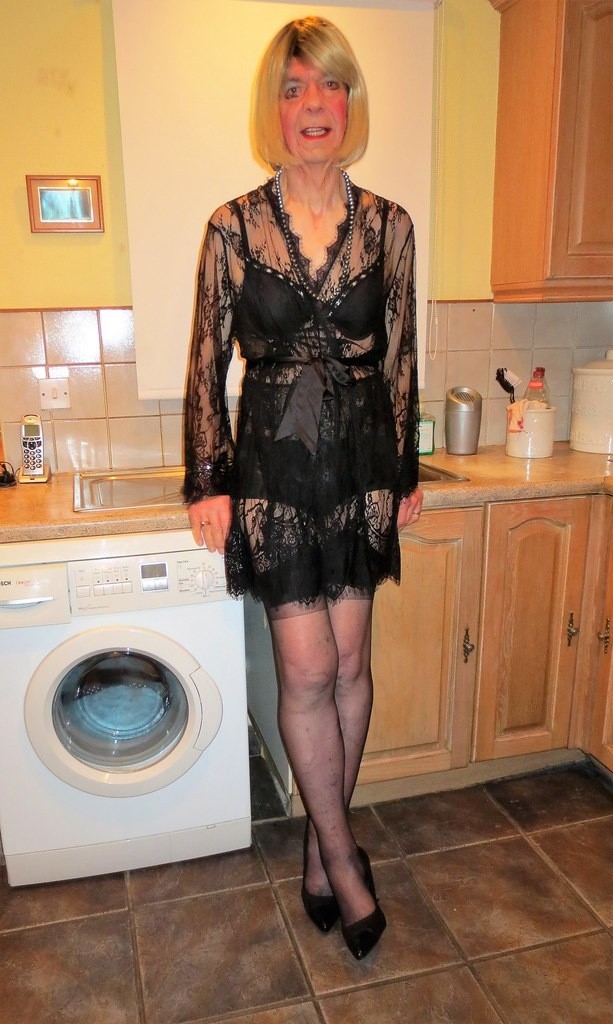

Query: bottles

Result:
[530,366,551,408]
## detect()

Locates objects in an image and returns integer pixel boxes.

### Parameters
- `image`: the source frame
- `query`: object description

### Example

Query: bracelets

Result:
[187,464,216,477]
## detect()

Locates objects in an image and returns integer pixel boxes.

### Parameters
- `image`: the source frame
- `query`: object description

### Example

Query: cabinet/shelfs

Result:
[491,0,613,305]
[243,492,612,821]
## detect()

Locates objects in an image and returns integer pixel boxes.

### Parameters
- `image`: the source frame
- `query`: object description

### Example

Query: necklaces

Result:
[276,170,355,302]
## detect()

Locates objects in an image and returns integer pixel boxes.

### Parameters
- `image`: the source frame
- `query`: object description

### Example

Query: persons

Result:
[182,16,420,960]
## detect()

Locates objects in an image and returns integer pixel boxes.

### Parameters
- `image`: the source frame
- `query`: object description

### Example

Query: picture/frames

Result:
[25,175,105,234]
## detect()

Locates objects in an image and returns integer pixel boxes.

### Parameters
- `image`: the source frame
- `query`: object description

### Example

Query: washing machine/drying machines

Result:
[0,526,254,889]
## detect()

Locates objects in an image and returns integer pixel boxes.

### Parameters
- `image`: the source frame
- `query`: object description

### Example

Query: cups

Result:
[569,349,613,455]
[505,403,556,459]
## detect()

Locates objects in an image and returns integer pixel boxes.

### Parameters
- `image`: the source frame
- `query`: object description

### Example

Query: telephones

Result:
[18,413,53,485]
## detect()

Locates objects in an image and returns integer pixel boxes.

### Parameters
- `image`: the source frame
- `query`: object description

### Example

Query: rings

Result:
[201,521,209,525]
[413,512,419,515]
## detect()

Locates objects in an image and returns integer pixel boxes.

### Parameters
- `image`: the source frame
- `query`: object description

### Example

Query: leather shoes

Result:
[341,846,386,961]
[301,815,340,933]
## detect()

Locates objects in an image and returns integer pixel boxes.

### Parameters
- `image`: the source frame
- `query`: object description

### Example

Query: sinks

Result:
[415,459,472,488]
